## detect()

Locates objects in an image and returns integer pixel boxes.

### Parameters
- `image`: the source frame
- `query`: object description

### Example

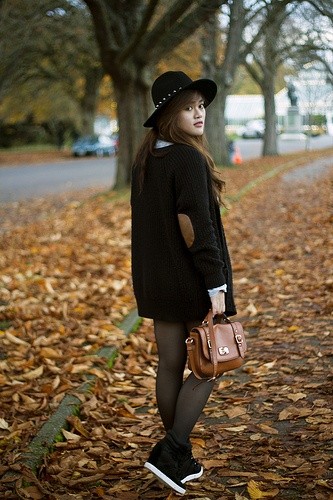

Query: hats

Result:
[141,70,219,129]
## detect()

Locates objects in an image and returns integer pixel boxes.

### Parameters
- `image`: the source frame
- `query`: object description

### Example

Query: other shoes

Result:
[142,433,187,496]
[178,452,205,484]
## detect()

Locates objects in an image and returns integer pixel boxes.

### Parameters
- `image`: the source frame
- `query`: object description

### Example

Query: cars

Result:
[71,134,118,157]
[240,121,280,139]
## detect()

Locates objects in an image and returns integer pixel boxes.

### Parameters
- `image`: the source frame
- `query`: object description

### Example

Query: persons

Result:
[129,69,257,495]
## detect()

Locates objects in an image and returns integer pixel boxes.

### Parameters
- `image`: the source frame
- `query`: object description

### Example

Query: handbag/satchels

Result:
[186,308,247,383]
